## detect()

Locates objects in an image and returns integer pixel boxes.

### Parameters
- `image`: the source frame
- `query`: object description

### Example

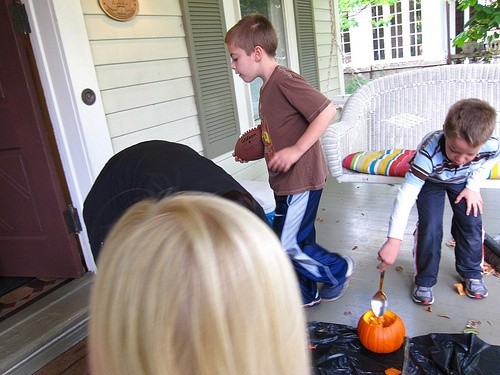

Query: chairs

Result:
[83,139,273,271]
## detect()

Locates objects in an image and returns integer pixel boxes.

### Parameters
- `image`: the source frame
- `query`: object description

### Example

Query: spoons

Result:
[371,269,388,318]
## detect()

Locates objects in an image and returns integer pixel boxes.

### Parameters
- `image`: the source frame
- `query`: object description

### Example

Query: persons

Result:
[377,98,497,304]
[87,191,316,375]
[225,13,353,308]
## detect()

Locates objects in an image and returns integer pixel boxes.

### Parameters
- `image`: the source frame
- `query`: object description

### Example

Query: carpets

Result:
[0,277,73,321]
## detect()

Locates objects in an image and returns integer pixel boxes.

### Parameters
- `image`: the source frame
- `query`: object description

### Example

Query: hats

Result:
[82,138,269,267]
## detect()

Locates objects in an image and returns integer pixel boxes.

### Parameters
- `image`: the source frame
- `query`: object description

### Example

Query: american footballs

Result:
[235,128,264,161]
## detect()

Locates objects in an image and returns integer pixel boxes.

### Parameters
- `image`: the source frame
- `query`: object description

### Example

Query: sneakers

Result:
[412,286,434,305]
[460,277,488,299]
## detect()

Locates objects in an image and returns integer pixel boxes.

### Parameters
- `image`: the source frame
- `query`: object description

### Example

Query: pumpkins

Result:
[357,308,405,354]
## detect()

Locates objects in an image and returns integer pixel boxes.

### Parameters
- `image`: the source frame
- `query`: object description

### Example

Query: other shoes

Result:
[320,256,355,301]
[303,291,320,310]
